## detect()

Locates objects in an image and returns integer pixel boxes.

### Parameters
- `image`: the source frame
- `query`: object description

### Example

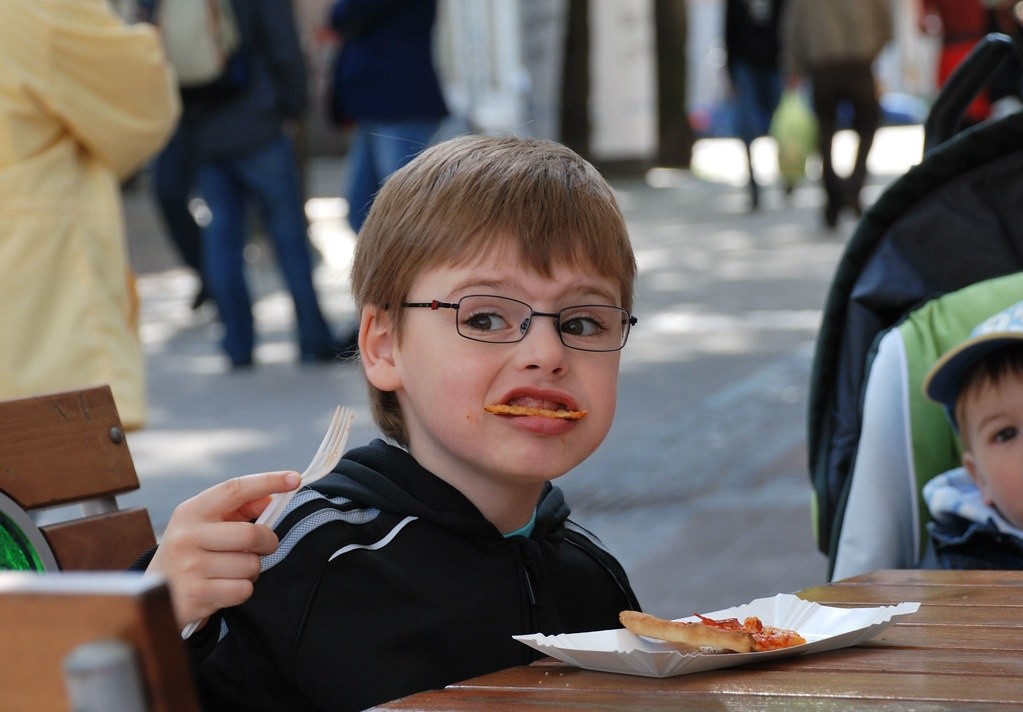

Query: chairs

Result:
[0,383,204,712]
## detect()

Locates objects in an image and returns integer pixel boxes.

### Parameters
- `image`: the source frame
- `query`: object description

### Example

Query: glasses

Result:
[381,294,638,353]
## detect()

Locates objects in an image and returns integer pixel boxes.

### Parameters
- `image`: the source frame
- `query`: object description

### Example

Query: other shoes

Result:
[299,340,345,362]
[740,160,881,228]
[227,345,251,367]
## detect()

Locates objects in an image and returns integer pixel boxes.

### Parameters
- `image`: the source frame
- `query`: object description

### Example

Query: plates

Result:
[512,593,921,677]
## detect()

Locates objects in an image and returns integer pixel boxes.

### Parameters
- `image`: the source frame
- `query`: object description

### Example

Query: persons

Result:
[917,302,1023,570]
[0,1,183,433]
[128,136,642,712]
[325,1,449,352]
[117,0,350,367]
[781,0,894,229]
[723,1,793,214]
[912,0,1023,140]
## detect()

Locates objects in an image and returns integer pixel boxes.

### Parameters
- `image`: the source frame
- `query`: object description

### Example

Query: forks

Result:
[181,405,355,642]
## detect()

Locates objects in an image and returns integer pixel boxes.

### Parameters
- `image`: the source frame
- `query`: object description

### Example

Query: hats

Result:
[920,302,1023,406]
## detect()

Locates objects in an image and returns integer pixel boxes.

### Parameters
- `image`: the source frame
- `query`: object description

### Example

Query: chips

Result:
[484,404,590,419]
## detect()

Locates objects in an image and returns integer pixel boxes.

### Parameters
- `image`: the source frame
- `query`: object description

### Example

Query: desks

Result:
[359,570,1023,711]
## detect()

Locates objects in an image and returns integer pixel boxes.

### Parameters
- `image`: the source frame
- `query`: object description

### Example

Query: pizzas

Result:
[618,607,807,653]
